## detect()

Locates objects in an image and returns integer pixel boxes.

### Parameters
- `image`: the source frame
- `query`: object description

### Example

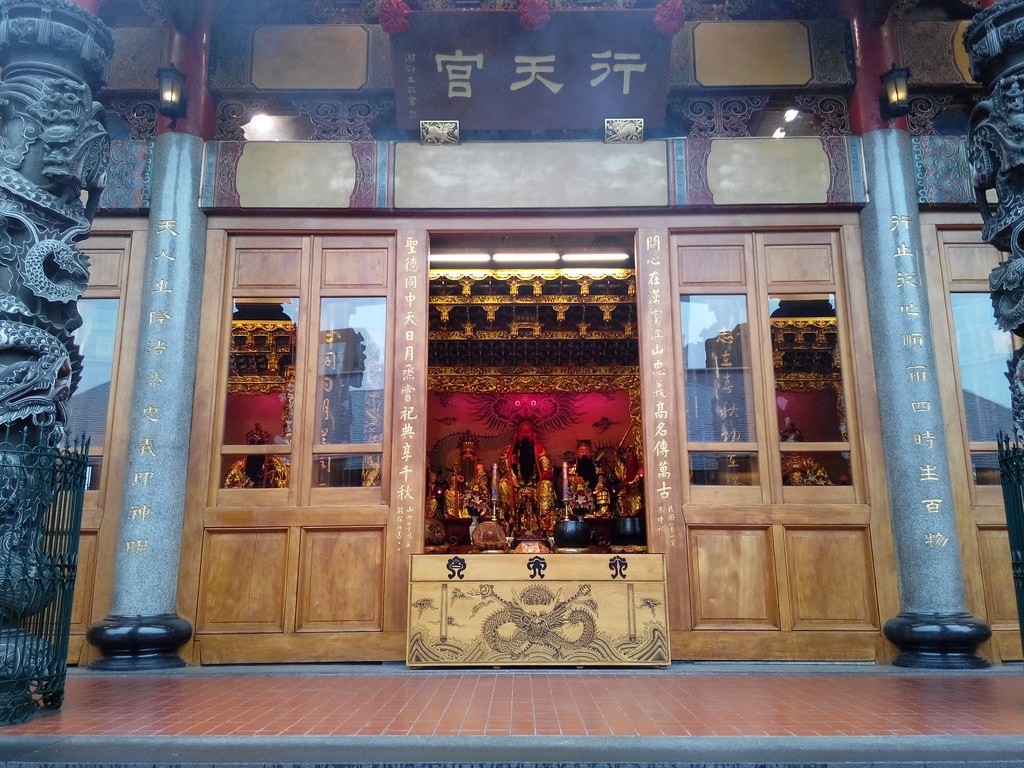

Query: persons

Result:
[615,447,644,515]
[446,441,490,518]
[782,423,833,486]
[224,423,279,488]
[568,438,611,517]
[498,420,556,515]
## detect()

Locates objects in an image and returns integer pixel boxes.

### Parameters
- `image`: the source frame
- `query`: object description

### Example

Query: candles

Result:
[492,463,498,498]
[563,462,567,498]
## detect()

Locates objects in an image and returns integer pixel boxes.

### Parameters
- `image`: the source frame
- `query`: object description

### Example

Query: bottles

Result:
[469,516,480,545]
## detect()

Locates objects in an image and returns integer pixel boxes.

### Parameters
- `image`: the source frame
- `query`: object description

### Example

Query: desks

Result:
[405,552,672,670]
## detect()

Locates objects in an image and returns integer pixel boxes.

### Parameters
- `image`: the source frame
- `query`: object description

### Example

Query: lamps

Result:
[153,62,188,130]
[878,63,910,120]
